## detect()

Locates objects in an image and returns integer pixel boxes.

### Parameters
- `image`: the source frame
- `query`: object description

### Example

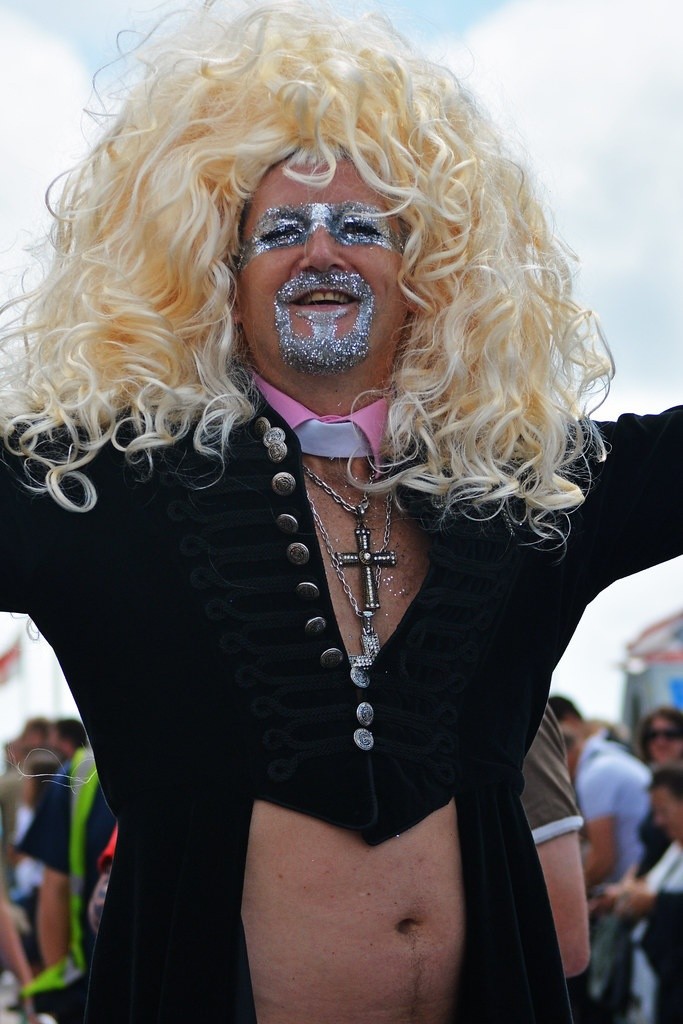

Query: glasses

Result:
[646,730,683,739]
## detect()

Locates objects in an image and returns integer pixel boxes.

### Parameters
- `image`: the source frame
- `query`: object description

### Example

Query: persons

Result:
[0,0,682,1024]
[1,694,681,1024]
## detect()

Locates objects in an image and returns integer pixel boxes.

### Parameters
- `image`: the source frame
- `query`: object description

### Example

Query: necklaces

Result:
[305,452,401,670]
